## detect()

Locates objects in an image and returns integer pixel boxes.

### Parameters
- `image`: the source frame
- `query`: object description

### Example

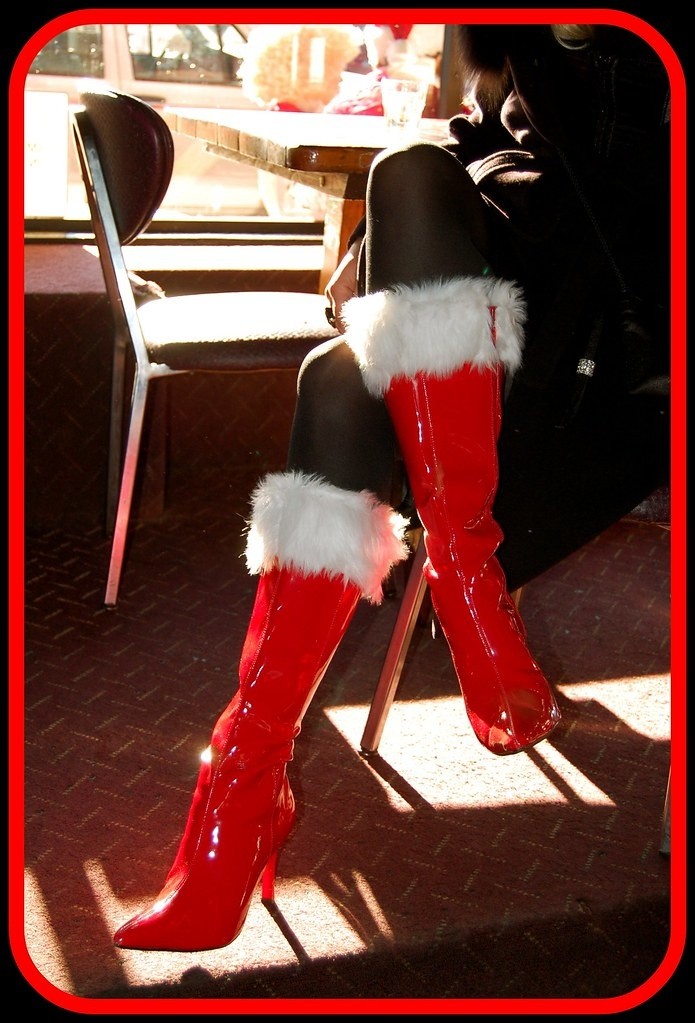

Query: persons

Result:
[113,22,674,954]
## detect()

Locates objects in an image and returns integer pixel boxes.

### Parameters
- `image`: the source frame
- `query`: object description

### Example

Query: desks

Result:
[166,109,475,293]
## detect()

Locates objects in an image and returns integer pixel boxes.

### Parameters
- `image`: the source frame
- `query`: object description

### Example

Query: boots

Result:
[341,278,562,756]
[112,470,409,952]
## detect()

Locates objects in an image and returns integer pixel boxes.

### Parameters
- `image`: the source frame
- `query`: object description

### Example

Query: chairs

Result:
[73,88,345,612]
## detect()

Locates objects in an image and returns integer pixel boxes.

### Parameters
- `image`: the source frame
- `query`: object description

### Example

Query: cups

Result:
[382,78,425,130]
